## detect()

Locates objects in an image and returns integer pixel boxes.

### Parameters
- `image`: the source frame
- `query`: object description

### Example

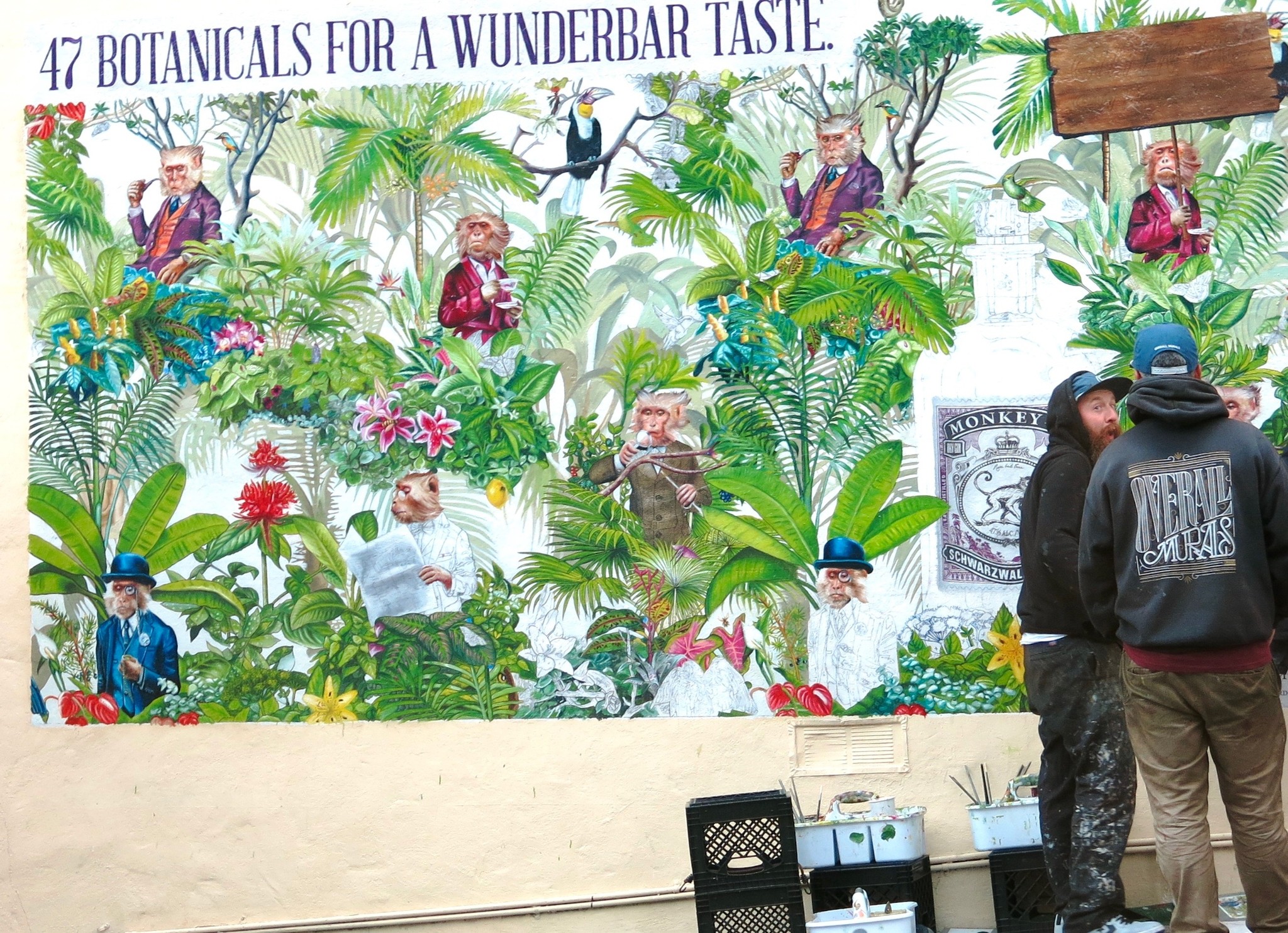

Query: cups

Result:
[869,797,895,816]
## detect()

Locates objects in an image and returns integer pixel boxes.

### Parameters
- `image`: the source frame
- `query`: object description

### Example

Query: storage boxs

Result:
[989,847,1056,933]
[685,790,800,892]
[809,854,936,933]
[694,885,806,933]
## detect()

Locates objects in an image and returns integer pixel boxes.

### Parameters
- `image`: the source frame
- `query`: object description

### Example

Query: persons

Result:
[1015,324,1288,933]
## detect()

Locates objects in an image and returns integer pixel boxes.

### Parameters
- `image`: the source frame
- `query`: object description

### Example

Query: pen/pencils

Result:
[949,775,979,805]
[964,765,982,805]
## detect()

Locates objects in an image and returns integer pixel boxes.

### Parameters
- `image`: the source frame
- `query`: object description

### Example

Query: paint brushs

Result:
[1016,761,1032,777]
[981,763,993,805]
[778,780,801,823]
[817,786,823,822]
[789,776,806,823]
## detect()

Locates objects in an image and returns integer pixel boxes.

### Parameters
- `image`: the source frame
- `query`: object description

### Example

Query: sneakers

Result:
[1054,914,1065,933]
[1087,915,1166,933]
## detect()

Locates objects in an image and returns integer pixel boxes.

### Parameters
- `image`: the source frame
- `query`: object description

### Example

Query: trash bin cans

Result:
[685,789,806,933]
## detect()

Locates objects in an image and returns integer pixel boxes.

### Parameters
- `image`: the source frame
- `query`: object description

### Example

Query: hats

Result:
[1130,323,1199,375]
[1070,371,1134,404]
